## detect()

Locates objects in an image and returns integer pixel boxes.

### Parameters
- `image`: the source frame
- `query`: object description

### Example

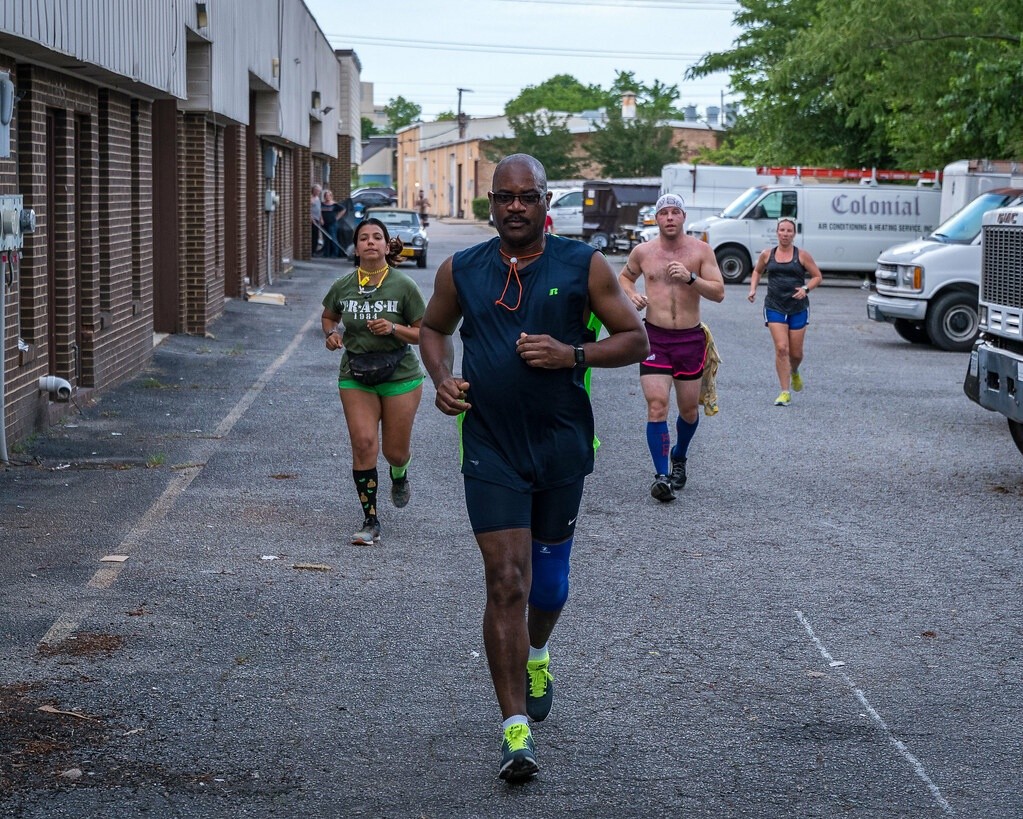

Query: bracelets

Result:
[391,323,396,335]
[802,286,810,294]
[326,328,340,338]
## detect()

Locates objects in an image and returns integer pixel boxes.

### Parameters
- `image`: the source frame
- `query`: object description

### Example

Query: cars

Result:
[365,209,433,269]
[350,186,398,207]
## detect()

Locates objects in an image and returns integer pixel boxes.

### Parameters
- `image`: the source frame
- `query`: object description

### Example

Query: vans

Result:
[489,163,818,266]
[863,188,1023,352]
[940,159,1023,230]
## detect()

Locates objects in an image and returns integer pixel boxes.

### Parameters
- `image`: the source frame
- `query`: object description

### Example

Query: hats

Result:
[654,194,685,214]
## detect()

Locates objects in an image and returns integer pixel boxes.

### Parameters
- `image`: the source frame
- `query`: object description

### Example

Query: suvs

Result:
[963,198,1023,456]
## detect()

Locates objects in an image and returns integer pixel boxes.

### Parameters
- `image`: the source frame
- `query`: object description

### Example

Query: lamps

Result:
[319,106,335,115]
[272,58,279,77]
[195,1,208,29]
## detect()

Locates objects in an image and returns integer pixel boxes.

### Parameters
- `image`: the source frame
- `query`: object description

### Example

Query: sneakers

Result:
[351,518,380,545]
[774,390,792,406]
[651,474,677,502]
[670,444,687,489]
[498,722,540,781]
[790,370,803,391]
[389,465,411,508]
[526,651,554,722]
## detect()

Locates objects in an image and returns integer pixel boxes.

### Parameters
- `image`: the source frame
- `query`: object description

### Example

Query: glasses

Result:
[489,189,546,206]
[778,216,796,223]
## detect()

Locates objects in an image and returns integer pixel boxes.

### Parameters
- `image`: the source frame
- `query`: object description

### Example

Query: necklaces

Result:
[358,265,391,295]
[494,247,544,310]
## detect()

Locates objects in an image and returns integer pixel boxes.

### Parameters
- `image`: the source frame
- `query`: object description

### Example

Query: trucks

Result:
[682,165,940,290]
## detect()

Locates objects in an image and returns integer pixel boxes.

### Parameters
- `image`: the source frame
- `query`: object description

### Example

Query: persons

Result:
[417,189,432,230]
[544,215,555,234]
[320,190,346,258]
[322,218,427,545]
[311,184,323,258]
[618,193,724,502]
[419,153,650,785]
[748,220,822,406]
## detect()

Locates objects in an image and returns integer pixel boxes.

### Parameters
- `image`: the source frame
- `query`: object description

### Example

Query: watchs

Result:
[570,343,585,369]
[685,272,697,286]
